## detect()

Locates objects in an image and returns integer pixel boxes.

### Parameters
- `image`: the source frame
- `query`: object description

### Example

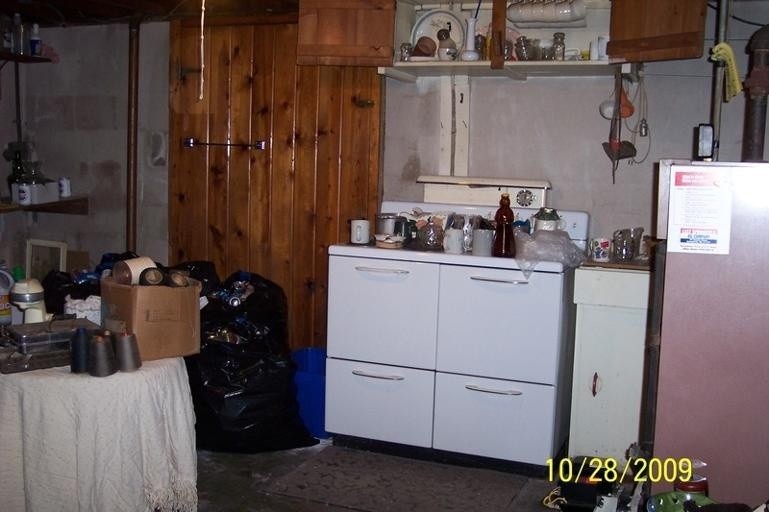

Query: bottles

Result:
[0,12,42,58]
[492,193,516,258]
[514,32,565,61]
[437,28,456,49]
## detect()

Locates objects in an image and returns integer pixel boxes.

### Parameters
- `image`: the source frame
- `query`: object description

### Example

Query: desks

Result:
[0,357,199,510]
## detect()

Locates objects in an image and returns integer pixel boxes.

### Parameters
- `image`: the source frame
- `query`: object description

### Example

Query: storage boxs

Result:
[98,275,202,363]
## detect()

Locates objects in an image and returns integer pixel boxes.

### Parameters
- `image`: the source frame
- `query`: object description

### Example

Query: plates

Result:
[409,56,435,61]
[410,9,465,59]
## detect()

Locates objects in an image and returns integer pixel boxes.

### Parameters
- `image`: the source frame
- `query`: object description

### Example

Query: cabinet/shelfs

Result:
[566,267,651,479]
[0,47,91,217]
[325,254,561,467]
[294,0,710,78]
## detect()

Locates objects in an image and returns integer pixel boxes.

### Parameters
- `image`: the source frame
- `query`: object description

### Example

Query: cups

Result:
[506,0,587,24]
[472,228,494,257]
[442,228,465,255]
[588,238,612,262]
[410,37,436,57]
[437,47,458,61]
[350,219,371,246]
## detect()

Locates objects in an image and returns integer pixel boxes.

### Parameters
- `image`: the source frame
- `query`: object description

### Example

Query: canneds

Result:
[674,473,708,499]
